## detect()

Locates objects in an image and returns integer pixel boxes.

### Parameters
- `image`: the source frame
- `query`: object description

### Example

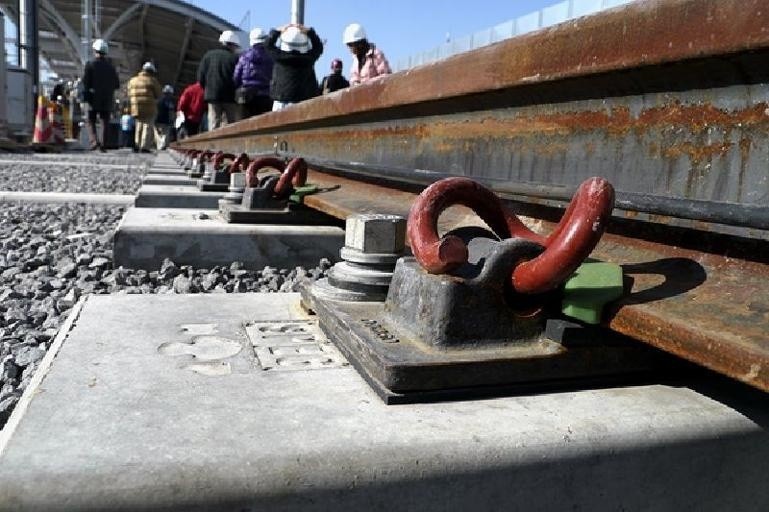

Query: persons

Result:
[177,27,325,137]
[320,60,350,92]
[50,80,64,100]
[65,81,78,139]
[79,38,120,152]
[155,83,177,148]
[344,23,391,84]
[125,61,163,152]
[121,107,134,147]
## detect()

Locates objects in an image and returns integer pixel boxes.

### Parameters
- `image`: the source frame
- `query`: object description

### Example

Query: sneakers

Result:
[89,140,105,153]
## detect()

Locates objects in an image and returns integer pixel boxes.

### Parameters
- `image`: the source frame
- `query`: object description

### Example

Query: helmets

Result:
[345,24,366,43]
[280,26,307,51]
[162,85,173,93]
[219,30,239,45]
[332,59,342,69]
[143,62,154,71]
[250,29,266,43]
[93,39,108,53]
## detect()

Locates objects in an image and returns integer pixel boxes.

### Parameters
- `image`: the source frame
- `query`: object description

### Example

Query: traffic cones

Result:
[31,91,68,153]
